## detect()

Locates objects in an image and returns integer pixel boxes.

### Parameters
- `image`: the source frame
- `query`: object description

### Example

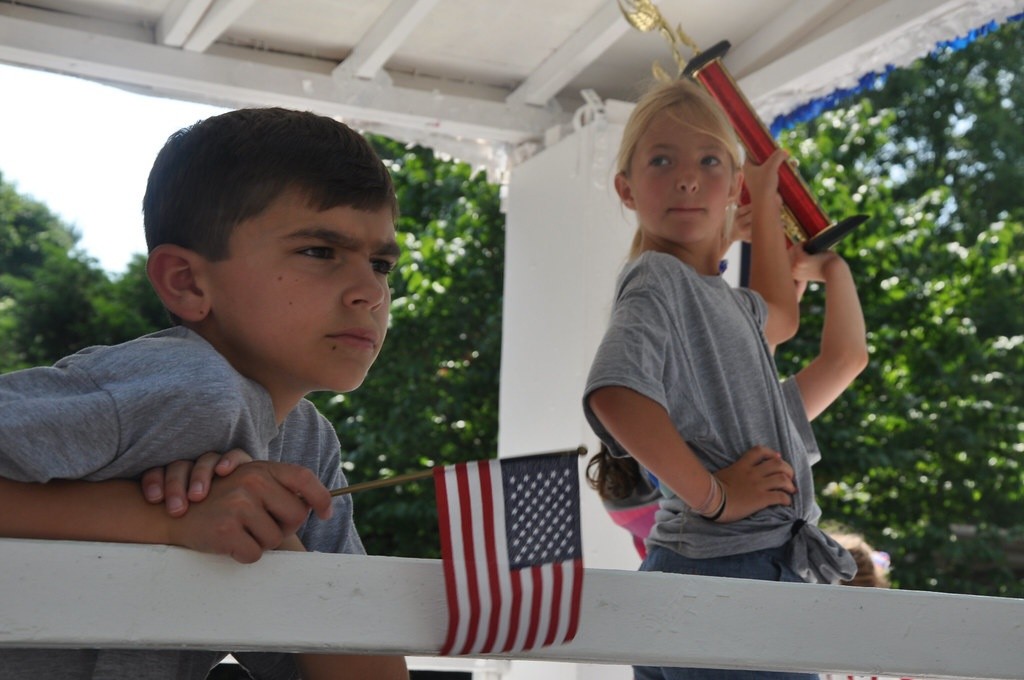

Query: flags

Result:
[433,447,583,660]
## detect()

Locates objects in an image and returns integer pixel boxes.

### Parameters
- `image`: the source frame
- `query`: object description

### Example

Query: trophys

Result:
[618,1,868,254]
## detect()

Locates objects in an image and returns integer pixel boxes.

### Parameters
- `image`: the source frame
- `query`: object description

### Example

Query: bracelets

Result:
[691,471,717,514]
[702,478,727,522]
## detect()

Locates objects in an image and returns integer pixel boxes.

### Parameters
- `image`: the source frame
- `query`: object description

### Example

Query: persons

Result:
[805,536,894,678]
[0,108,414,676]
[588,202,870,563]
[582,77,859,680]
